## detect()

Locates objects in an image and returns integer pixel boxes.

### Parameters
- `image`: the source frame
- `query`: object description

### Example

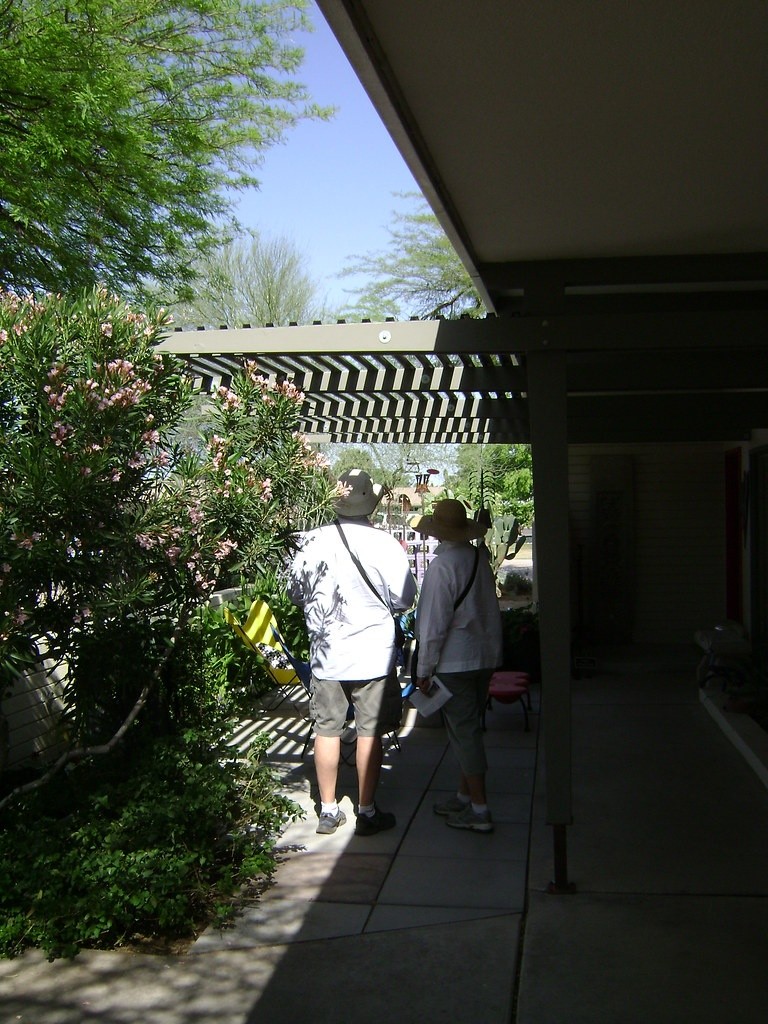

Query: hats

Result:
[328,468,384,517]
[411,498,489,543]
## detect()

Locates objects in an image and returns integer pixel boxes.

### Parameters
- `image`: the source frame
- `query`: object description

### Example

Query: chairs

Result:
[268,621,403,769]
[223,600,311,722]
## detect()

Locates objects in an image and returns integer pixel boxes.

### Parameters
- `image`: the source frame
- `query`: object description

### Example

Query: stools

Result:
[481,672,534,733]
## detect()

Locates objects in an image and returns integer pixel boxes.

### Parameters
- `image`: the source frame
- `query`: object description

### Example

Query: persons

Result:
[290,466,417,836]
[411,498,508,830]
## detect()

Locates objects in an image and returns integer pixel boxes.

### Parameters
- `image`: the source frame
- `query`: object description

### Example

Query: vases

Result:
[503,631,542,683]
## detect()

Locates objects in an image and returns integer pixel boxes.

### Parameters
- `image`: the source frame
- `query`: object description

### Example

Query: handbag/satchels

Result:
[394,616,406,648]
[409,642,437,687]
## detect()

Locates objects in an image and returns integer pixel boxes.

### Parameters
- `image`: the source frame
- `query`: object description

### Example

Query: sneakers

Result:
[433,791,488,815]
[315,805,348,834]
[445,803,493,830]
[354,805,397,836]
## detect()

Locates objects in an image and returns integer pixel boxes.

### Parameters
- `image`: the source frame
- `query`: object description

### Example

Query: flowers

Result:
[500,602,540,645]
